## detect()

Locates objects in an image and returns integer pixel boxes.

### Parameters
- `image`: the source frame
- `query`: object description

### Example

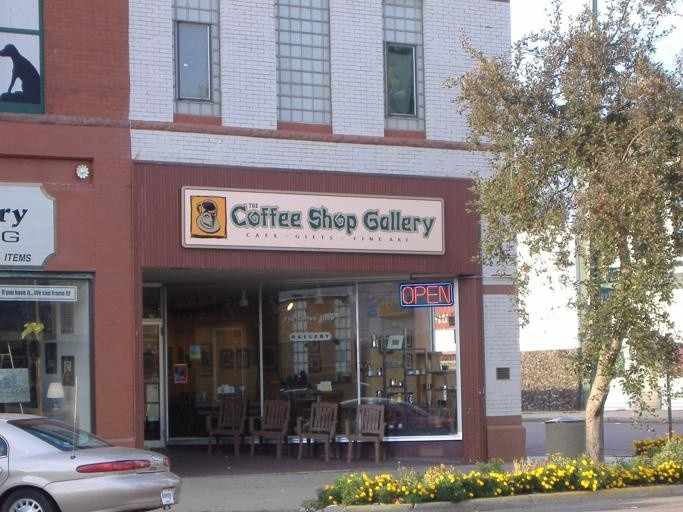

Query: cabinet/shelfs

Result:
[360,315,456,435]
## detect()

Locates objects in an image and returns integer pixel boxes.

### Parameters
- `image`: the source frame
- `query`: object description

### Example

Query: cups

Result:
[377,367,382,376]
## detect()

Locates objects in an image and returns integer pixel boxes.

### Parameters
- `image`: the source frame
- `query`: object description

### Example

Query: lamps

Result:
[46,382,64,412]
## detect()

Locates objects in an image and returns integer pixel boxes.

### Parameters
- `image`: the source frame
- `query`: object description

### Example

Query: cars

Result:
[294,398,455,436]
[0,413,182,512]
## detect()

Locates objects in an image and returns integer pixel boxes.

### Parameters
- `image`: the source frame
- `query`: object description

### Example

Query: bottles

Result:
[367,362,373,377]
[369,332,377,347]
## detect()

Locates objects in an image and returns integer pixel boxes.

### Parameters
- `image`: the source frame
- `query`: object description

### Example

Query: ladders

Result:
[0,343,23,414]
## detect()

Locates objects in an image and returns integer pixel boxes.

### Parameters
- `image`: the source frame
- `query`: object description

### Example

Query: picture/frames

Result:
[219,348,249,369]
[198,343,212,377]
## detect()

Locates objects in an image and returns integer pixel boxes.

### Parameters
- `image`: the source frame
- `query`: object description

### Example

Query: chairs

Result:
[205,396,385,464]
